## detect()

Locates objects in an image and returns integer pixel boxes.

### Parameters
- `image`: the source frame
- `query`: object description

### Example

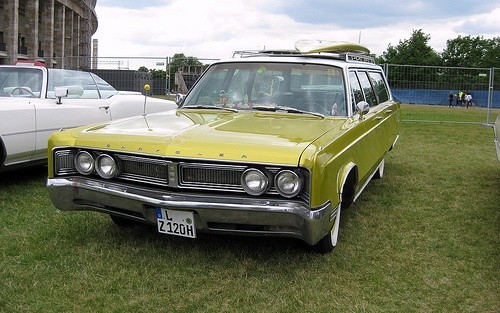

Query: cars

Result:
[46,38,402,256]
[0,61,180,186]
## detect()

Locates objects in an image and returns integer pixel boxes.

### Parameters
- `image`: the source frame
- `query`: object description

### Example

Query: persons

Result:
[454,91,472,108]
[448,90,454,108]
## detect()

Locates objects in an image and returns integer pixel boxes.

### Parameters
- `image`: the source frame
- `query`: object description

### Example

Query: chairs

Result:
[4,85,83,99]
